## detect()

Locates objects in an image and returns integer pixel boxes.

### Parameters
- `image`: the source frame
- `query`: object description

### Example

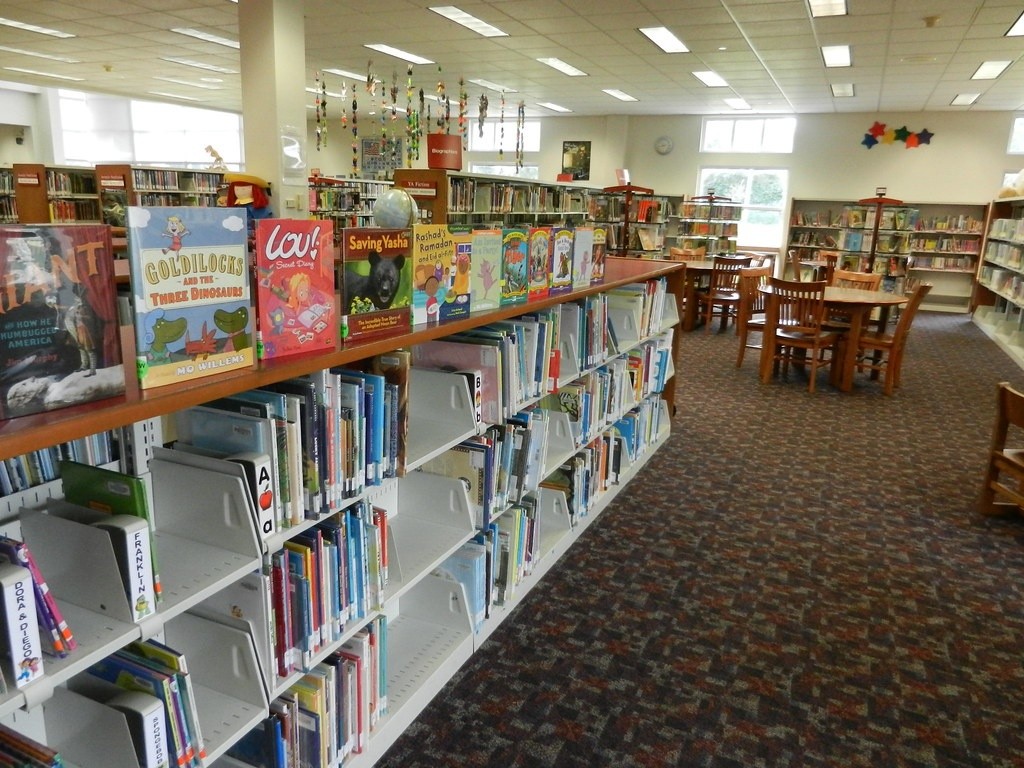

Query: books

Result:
[2,167,743,768]
[786,207,1024,302]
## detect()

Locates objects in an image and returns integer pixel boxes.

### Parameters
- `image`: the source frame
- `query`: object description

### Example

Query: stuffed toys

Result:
[223,172,274,239]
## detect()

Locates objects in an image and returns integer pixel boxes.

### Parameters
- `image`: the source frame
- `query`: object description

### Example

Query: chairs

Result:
[981,381,1024,537]
[639,247,933,395]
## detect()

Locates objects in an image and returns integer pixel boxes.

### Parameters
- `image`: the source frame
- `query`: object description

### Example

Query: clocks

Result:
[655,136,672,155]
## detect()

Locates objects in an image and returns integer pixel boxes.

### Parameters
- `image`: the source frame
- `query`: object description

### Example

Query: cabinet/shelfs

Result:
[780,197,989,314]
[0,161,744,768]
[971,197,1024,372]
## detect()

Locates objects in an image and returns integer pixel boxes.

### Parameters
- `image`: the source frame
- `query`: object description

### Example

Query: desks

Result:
[681,260,739,320]
[756,286,910,386]
